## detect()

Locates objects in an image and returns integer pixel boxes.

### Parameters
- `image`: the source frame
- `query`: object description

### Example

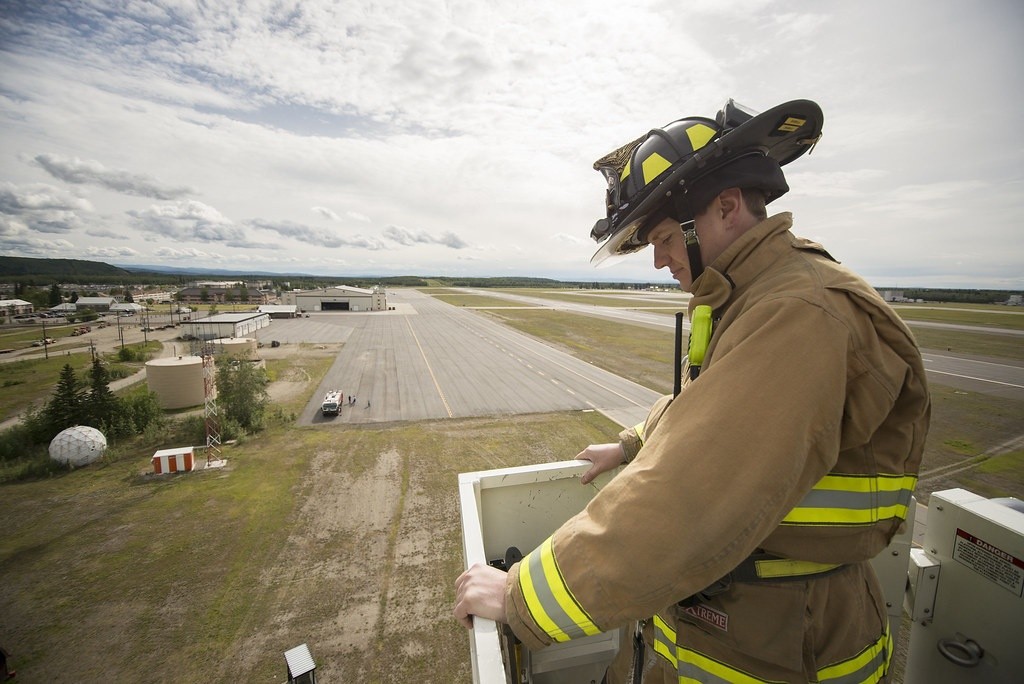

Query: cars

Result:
[13,311,75,319]
[141,324,176,332]
[71,327,87,337]
[32,337,53,348]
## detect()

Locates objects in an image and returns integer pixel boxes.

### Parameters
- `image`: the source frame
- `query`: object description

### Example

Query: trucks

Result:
[320,389,344,417]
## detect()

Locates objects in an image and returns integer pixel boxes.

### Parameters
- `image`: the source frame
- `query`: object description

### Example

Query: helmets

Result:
[589,98,824,268]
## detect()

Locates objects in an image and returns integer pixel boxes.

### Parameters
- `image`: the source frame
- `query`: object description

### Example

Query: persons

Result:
[348,395,356,405]
[367,400,370,407]
[452,98,931,684]
